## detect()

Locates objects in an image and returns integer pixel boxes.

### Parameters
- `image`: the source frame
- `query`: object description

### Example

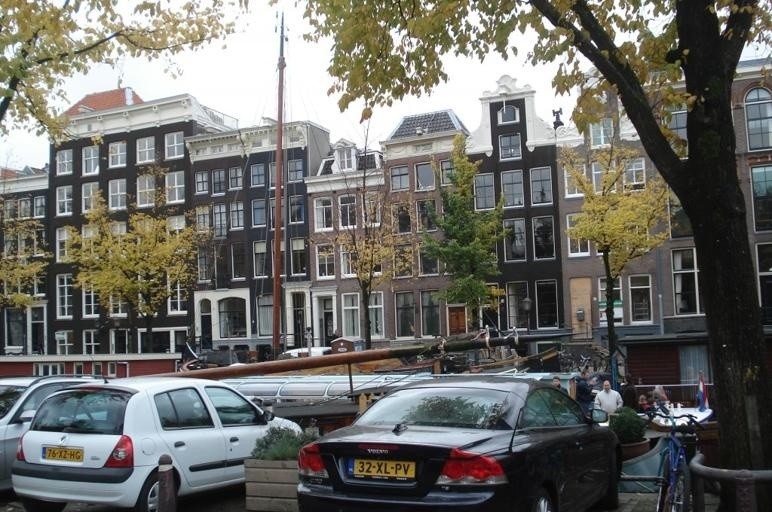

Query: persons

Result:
[550,370,668,421]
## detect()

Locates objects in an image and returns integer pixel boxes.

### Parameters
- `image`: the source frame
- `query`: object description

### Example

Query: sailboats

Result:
[176,12,588,377]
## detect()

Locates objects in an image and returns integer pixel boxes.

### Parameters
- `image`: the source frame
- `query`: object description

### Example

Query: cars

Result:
[1,374,106,491]
[294,375,626,511]
[12,375,306,512]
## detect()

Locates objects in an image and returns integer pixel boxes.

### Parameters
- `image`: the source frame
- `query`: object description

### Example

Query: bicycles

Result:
[642,406,705,512]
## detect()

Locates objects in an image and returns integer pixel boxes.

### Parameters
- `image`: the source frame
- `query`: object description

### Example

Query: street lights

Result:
[521,297,531,336]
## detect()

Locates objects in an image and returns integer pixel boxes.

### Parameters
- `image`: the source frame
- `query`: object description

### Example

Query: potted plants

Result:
[611,409,649,459]
[241,426,320,511]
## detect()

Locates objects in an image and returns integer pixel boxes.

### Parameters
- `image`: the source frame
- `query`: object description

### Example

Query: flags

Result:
[695,375,710,413]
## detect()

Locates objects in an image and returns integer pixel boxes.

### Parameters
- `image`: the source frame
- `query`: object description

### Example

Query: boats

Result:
[144,330,714,430]
